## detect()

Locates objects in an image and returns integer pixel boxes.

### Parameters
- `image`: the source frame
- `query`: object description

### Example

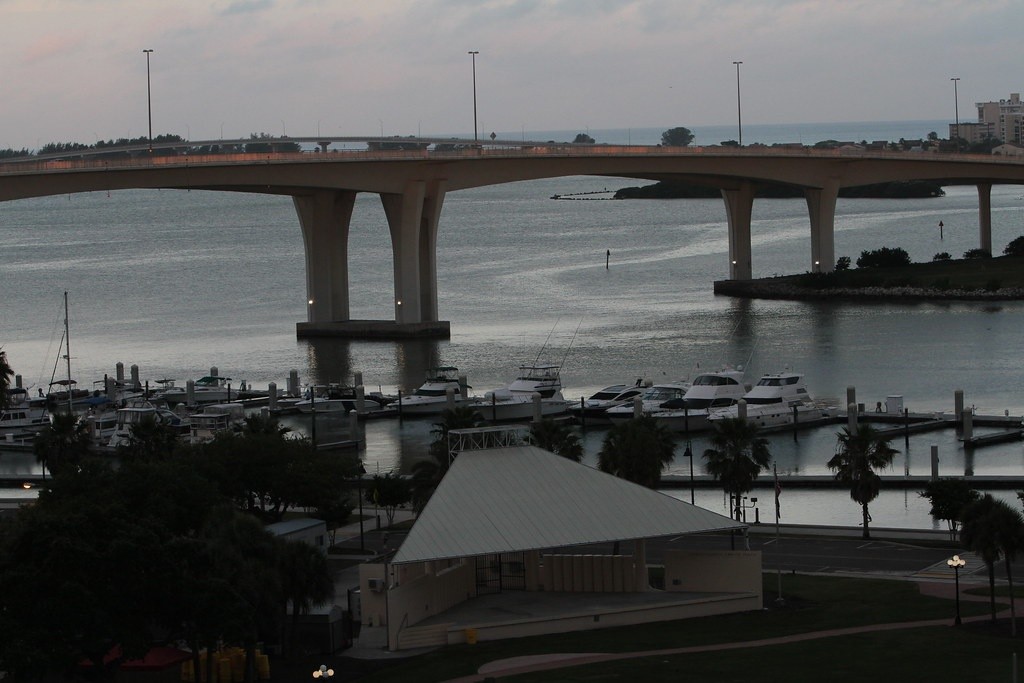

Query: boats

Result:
[0,290,396,448]
[386,351,485,413]
[565,376,647,428]
[647,316,762,434]
[704,365,836,434]
[469,316,586,422]
[602,372,693,430]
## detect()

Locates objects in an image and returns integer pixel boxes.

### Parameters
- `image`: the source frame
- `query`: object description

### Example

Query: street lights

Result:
[142,49,154,147]
[467,51,480,144]
[733,61,743,150]
[947,554,967,625]
[683,439,695,505]
[950,77,962,154]
[356,456,368,554]
